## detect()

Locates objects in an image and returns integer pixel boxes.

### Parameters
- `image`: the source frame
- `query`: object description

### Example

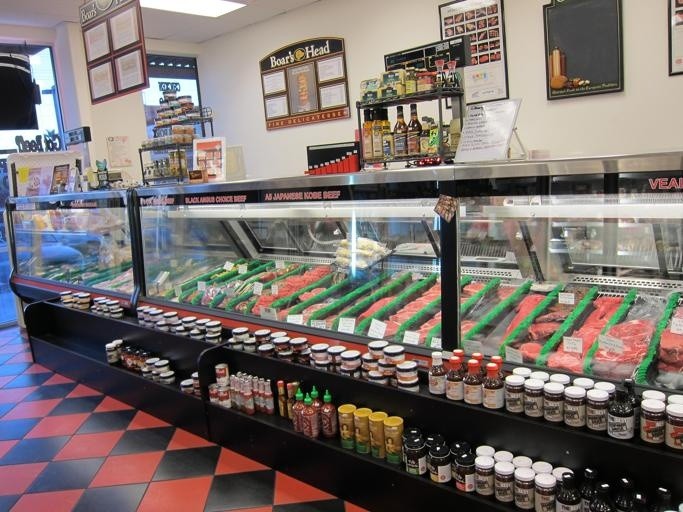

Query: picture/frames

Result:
[192,134,226,183]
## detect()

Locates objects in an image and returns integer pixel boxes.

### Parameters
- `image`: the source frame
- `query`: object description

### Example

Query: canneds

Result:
[416,72,436,94]
[105,338,683,512]
[61,291,287,345]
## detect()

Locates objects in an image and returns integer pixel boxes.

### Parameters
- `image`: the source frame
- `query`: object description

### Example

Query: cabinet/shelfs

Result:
[0,150,681,512]
[135,143,194,187]
[149,114,214,151]
[356,82,464,175]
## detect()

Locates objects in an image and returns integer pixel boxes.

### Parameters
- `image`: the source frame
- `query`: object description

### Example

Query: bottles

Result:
[555,463,683,511]
[296,75,308,107]
[228,371,299,417]
[394,105,409,160]
[407,103,423,153]
[434,58,459,88]
[168,149,188,176]
[292,386,337,439]
[361,108,389,160]
[152,158,167,177]
[120,346,152,372]
[605,378,641,440]
[418,123,430,153]
[404,64,427,93]
[428,348,504,412]
